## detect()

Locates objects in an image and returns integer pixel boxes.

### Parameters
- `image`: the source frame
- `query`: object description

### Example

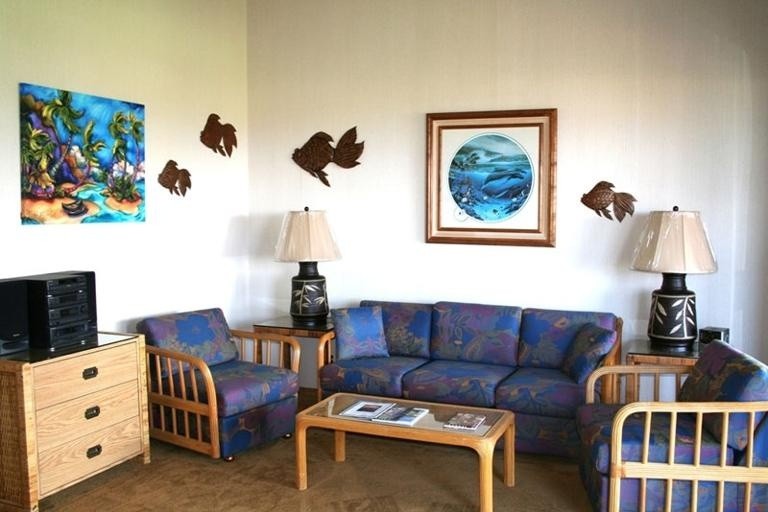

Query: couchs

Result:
[316,299,624,460]
[575,339,767,512]
[136,307,302,465]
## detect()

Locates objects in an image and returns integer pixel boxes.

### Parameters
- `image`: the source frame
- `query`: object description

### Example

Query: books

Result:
[337,399,488,429]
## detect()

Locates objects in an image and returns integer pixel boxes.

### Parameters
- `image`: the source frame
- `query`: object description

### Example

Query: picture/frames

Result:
[425,106,559,249]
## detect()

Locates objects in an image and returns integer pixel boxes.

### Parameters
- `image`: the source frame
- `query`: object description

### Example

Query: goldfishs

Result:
[291,125,365,187]
[580,181,637,224]
[199,113,237,158]
[157,159,191,196]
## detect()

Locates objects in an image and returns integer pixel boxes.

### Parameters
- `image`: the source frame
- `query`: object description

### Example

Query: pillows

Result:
[558,321,617,384]
[330,304,392,363]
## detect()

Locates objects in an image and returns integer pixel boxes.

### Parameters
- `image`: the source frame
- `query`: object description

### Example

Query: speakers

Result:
[0,278,30,356]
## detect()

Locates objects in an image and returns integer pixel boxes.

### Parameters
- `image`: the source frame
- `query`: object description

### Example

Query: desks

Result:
[251,315,335,401]
[624,333,706,404]
[0,330,153,512]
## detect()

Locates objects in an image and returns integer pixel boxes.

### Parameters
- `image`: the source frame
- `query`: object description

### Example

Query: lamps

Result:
[273,204,341,327]
[627,204,724,355]
